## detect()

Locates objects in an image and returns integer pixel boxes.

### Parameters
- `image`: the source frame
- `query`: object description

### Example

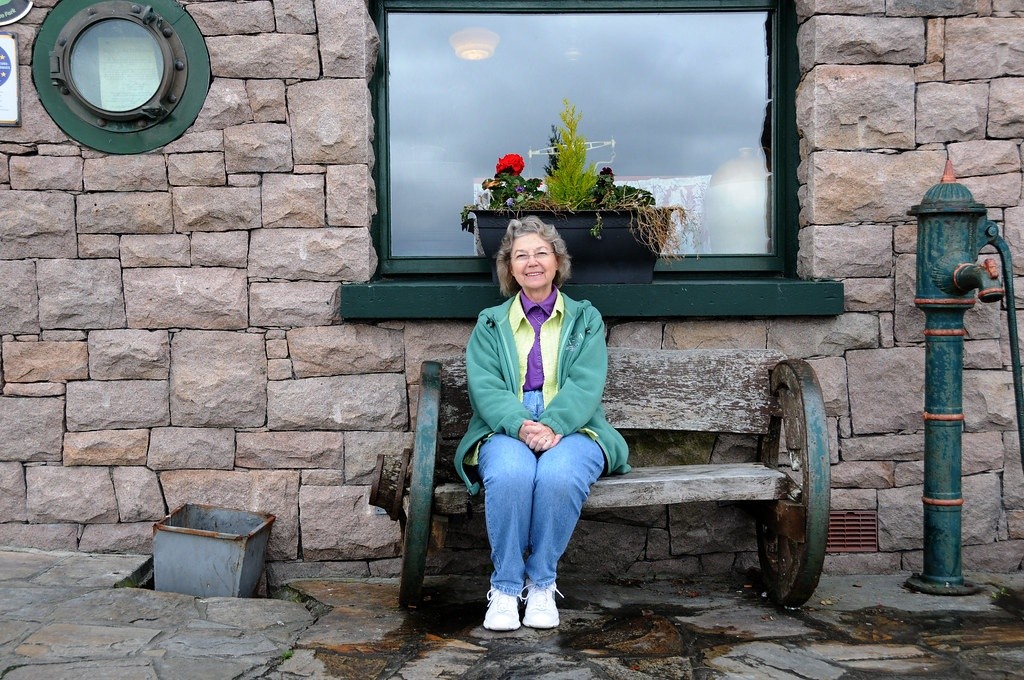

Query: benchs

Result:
[368,346,832,610]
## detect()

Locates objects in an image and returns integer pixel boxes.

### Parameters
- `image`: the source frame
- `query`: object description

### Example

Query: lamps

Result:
[448,27,501,60]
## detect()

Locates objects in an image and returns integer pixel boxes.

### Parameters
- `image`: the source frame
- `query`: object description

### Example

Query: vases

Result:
[468,209,673,284]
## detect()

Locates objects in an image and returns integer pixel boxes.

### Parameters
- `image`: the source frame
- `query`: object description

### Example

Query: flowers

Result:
[460,95,703,266]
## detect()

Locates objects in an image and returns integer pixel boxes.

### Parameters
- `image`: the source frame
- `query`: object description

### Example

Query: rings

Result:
[542,437,547,441]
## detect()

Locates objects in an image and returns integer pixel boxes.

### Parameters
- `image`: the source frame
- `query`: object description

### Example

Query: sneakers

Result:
[483,584,522,630]
[519,579,564,628]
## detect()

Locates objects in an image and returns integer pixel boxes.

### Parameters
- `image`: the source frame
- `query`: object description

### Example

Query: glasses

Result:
[510,251,555,264]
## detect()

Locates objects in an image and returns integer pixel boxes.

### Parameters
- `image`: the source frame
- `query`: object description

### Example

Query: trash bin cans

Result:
[148,501,277,598]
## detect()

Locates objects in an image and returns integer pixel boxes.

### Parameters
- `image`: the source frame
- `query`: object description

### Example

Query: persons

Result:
[452,216,628,631]
